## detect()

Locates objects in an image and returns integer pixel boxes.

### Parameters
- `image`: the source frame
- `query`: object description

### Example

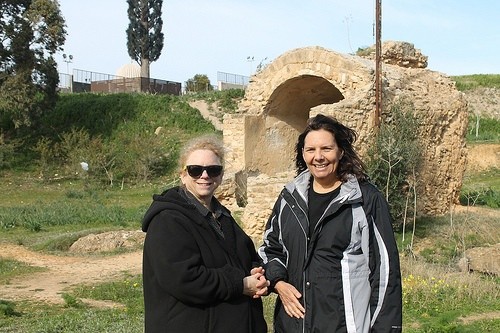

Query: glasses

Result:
[183,164,224,178]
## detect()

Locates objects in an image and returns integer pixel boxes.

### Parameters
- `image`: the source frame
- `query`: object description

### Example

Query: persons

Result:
[140,132,274,333]
[260,113,403,333]
